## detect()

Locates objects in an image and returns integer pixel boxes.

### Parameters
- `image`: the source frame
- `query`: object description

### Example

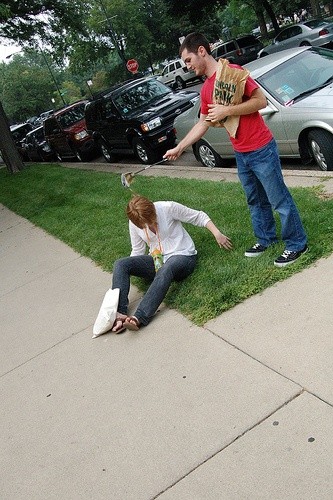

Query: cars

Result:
[256,17,333,59]
[142,56,180,77]
[156,59,206,91]
[172,46,333,171]
[9,106,67,162]
[252,4,332,35]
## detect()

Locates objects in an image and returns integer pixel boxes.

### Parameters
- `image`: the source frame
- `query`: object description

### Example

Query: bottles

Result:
[152,248,163,272]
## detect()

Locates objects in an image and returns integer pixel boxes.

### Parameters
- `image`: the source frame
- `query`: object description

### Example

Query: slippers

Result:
[123,317,140,330]
[113,318,126,334]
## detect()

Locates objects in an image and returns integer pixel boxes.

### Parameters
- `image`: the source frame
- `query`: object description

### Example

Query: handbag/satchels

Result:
[92,288,120,339]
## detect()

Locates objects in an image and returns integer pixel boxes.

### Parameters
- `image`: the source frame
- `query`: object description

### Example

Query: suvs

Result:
[85,77,200,165]
[211,34,266,66]
[42,99,102,162]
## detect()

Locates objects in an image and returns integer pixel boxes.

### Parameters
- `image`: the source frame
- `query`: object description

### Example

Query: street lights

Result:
[6,43,66,109]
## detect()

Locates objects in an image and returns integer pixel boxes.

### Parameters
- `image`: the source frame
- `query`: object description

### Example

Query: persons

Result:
[111,196,233,334]
[162,33,308,266]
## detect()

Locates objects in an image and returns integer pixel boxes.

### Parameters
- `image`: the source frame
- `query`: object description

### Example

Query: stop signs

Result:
[127,58,140,73]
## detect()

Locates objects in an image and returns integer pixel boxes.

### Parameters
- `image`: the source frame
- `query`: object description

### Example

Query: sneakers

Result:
[245,244,267,257]
[274,245,308,267]
